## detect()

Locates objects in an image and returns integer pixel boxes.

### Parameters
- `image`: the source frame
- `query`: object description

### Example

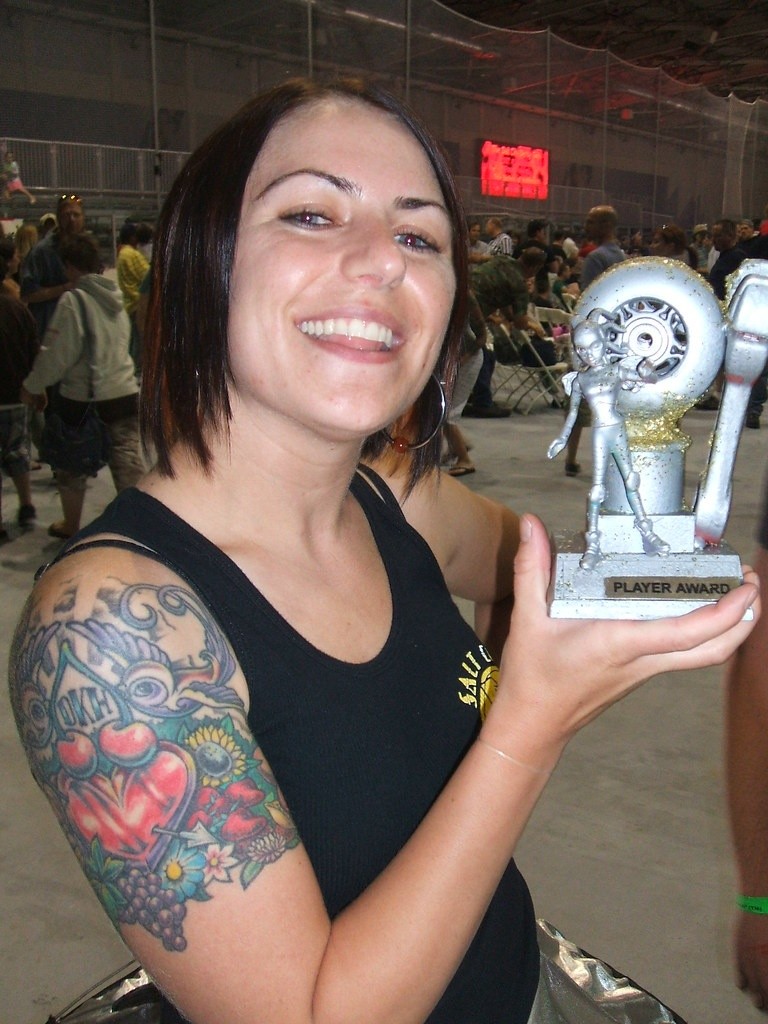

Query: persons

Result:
[720,470,766,1021]
[0,150,768,560]
[0,75,767,1024]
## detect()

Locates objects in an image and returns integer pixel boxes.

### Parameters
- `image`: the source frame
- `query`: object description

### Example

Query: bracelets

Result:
[733,894,767,916]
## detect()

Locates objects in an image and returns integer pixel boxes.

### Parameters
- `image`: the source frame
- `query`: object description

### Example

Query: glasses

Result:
[58,193,81,202]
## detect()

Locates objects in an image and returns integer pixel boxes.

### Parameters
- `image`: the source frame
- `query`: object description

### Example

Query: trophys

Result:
[545,256,768,622]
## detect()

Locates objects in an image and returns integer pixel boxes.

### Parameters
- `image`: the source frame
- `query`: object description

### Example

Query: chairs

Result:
[491,273,578,416]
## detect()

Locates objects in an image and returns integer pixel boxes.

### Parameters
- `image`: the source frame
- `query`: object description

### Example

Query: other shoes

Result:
[30,198,36,205]
[550,395,569,409]
[16,504,36,520]
[0,529,7,539]
[448,468,475,476]
[694,397,719,409]
[564,461,580,476]
[463,401,512,419]
[747,415,760,429]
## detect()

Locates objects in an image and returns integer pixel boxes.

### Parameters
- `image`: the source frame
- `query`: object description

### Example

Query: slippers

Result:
[48,523,72,539]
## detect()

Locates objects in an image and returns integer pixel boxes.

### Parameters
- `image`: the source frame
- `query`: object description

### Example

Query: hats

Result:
[693,224,707,235]
[40,213,57,226]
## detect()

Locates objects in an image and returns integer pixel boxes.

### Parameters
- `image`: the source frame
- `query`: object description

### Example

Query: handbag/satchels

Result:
[522,334,556,367]
[38,412,113,476]
[492,335,522,365]
